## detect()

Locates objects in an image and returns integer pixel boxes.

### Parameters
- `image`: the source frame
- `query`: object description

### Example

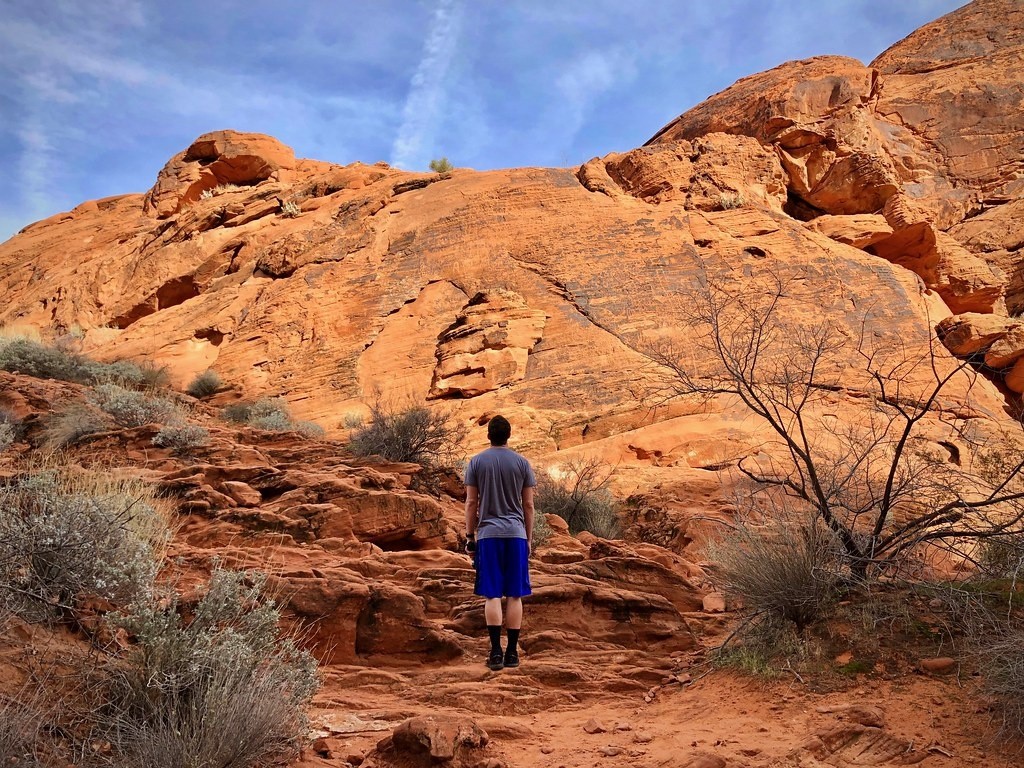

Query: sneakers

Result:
[486,655,520,670]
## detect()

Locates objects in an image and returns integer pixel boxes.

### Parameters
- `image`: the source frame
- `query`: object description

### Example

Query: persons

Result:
[464,414,537,670]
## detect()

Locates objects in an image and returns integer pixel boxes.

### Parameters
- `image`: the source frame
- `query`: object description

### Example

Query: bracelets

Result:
[466,533,475,538]
